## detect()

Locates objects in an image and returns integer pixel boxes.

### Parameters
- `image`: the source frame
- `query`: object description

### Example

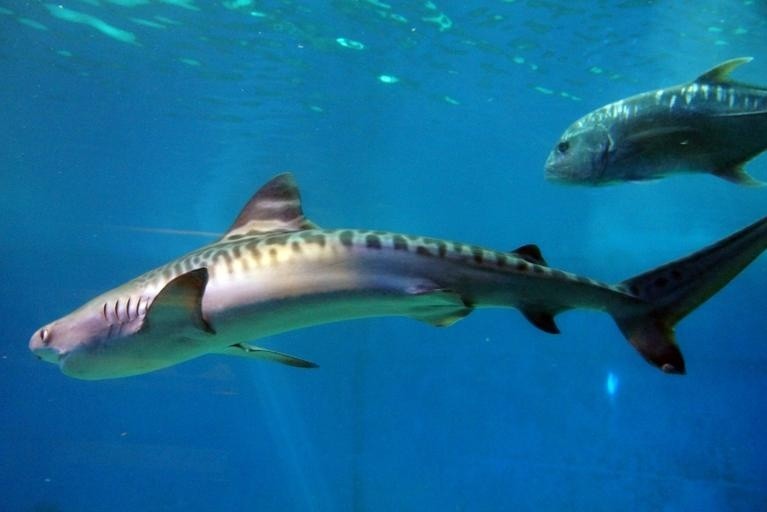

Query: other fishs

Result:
[541,55,767,191]
